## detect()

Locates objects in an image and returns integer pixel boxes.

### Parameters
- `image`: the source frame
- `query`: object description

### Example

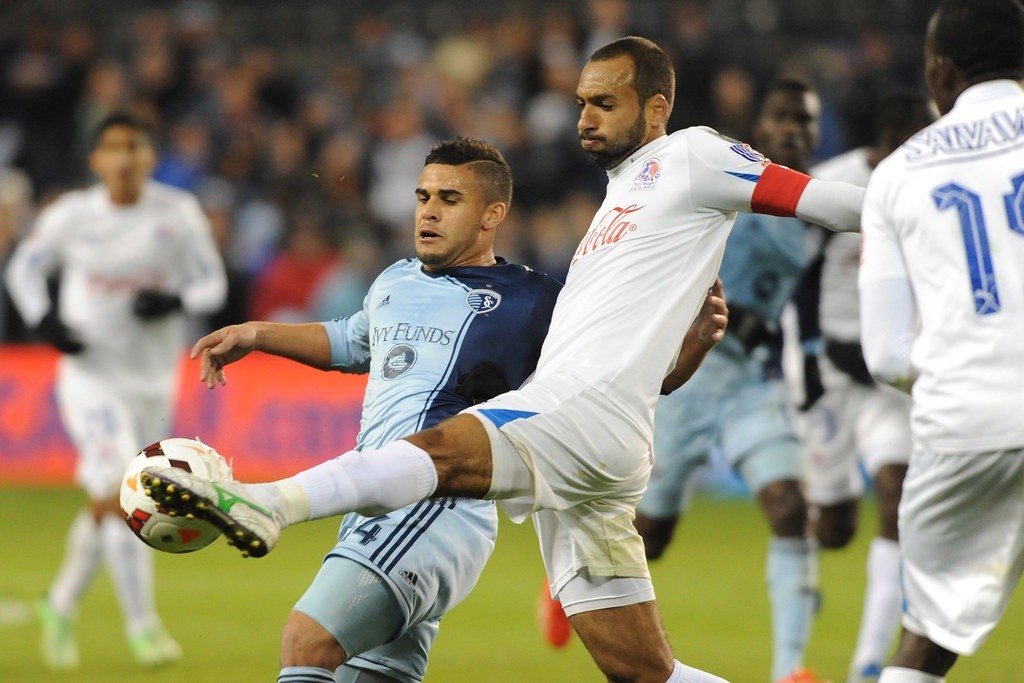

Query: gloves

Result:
[33,312,84,354]
[132,289,183,321]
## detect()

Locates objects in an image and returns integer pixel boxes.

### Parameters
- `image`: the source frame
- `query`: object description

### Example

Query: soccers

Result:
[119,437,234,554]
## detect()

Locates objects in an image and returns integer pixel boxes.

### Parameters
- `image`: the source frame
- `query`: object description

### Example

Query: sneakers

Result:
[140,466,283,558]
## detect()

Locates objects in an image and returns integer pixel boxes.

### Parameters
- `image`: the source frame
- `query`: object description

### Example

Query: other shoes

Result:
[125,620,182,667]
[36,590,80,671]
[779,670,820,683]
[539,575,573,648]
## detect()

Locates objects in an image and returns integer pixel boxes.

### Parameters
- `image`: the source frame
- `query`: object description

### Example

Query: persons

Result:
[859,0,1023,683]
[187,133,730,683]
[434,0,934,286]
[775,92,939,683]
[538,73,822,683]
[4,108,224,667]
[144,37,867,683]
[0,0,443,341]
